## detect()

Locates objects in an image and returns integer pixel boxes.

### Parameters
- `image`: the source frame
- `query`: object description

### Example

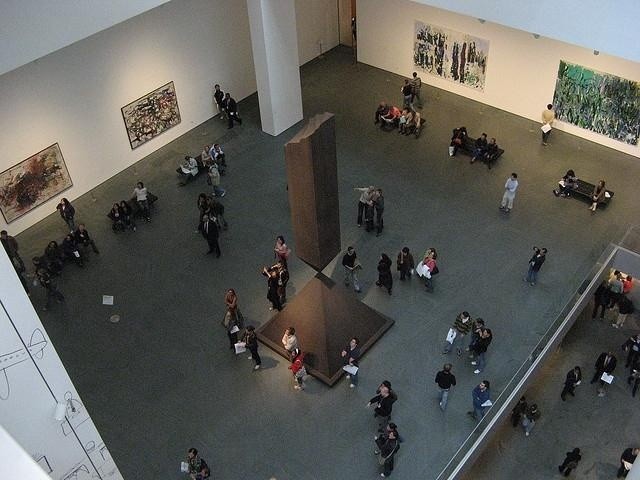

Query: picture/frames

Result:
[0,142,73,223]
[121,81,181,149]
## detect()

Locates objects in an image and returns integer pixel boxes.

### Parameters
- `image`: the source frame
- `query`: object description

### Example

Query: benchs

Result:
[176,155,212,187]
[375,110,425,139]
[553,179,614,208]
[450,136,504,169]
[39,242,69,273]
[107,191,158,232]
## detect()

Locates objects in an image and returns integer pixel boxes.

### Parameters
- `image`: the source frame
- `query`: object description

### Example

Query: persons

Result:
[499,172,519,212]
[182,447,210,480]
[341,245,439,296]
[351,16,355,40]
[448,126,497,165]
[214,84,241,130]
[339,337,360,389]
[588,180,607,213]
[354,184,386,237]
[366,310,492,478]
[0,182,151,311]
[541,103,555,146]
[510,270,640,479]
[195,214,221,259]
[373,72,423,139]
[524,246,547,286]
[553,169,579,200]
[222,235,308,393]
[179,141,227,234]
